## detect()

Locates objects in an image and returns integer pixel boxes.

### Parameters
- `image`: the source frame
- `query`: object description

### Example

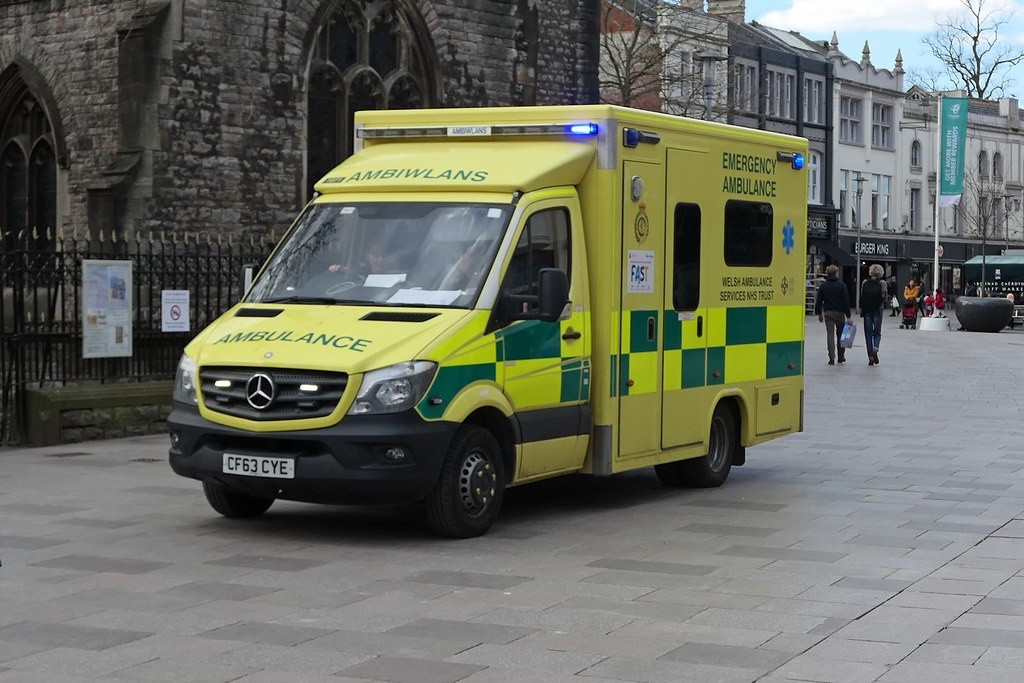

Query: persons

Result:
[934,289,947,318]
[916,282,926,317]
[887,275,902,317]
[925,291,936,317]
[329,233,407,300]
[976,282,990,298]
[904,280,920,329]
[815,265,855,365]
[957,279,977,331]
[859,264,888,365]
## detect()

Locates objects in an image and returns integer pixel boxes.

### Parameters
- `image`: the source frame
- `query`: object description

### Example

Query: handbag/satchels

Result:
[916,295,922,302]
[893,297,899,307]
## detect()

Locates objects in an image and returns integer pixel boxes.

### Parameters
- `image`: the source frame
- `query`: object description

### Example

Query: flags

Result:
[937,95,969,208]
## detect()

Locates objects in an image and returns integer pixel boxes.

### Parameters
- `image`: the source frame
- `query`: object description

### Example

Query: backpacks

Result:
[861,277,882,312]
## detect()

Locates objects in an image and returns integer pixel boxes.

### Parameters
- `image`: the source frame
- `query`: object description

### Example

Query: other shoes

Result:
[873,352,879,364]
[838,358,846,363]
[957,327,965,331]
[889,314,896,316]
[828,360,834,365]
[897,310,901,316]
[869,359,874,365]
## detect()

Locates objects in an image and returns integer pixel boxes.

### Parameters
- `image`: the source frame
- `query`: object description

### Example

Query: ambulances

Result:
[167,104,810,538]
